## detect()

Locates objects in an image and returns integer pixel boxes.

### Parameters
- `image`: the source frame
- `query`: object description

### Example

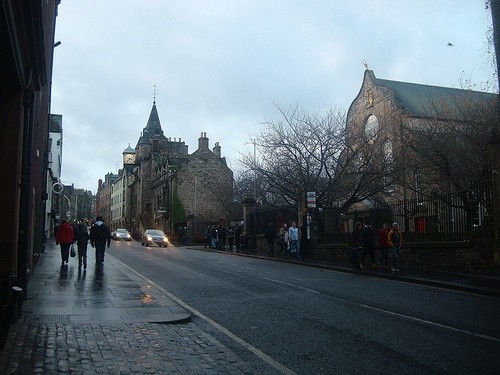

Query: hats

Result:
[392,223,398,227]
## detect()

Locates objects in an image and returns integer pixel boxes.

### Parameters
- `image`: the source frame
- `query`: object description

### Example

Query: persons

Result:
[276,223,288,257]
[56,218,74,264]
[378,223,391,267]
[218,224,240,249]
[177,225,187,245]
[74,222,90,269]
[90,216,111,265]
[285,222,298,257]
[360,220,376,269]
[352,222,363,268]
[388,222,402,272]
[264,223,277,255]
[204,224,216,249]
[80,219,86,225]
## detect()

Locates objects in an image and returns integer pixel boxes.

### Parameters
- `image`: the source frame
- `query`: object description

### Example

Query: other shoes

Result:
[371,263,377,269]
[384,264,390,267]
[359,262,365,269]
[391,267,400,272]
[379,259,383,266]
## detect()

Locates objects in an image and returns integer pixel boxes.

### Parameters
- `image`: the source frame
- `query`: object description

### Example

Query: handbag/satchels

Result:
[278,239,284,245]
[70,245,76,257]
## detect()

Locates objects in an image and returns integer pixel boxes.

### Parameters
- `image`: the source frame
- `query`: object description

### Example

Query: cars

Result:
[140,229,169,248]
[112,228,132,242]
